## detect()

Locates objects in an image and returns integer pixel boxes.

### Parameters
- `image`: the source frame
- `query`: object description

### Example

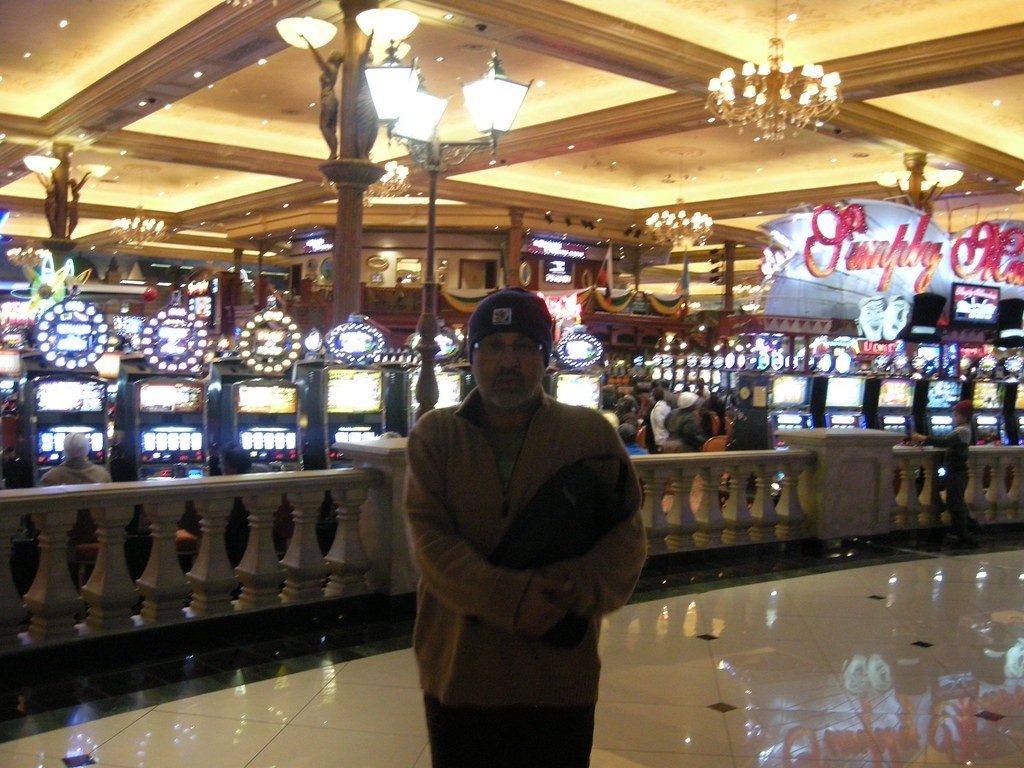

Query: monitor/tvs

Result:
[950,282,1000,329]
[771,415,1024,450]
[139,423,204,464]
[543,259,573,285]
[330,422,381,460]
[37,423,106,467]
[237,422,298,462]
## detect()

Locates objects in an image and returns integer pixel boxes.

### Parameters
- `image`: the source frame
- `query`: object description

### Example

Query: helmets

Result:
[64,432,88,457]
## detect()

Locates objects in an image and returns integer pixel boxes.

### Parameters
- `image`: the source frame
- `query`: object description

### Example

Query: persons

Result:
[400,284,648,768]
[30,429,120,571]
[0,431,24,497]
[910,398,989,554]
[603,367,776,452]
[207,440,255,474]
[645,380,672,453]
[665,387,705,452]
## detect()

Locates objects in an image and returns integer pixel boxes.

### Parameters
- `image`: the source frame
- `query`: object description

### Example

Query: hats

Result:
[678,392,700,409]
[467,287,551,369]
[952,400,974,420]
[618,424,636,439]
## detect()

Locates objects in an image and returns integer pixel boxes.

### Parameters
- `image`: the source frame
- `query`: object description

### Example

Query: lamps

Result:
[65,164,111,240]
[545,210,602,230]
[704,0,844,153]
[874,165,965,216]
[355,8,419,159]
[644,148,712,247]
[22,155,61,240]
[319,160,409,206]
[277,17,343,161]
[112,205,164,248]
[623,226,642,238]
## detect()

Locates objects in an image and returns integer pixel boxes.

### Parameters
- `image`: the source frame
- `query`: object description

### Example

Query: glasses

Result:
[473,336,544,356]
